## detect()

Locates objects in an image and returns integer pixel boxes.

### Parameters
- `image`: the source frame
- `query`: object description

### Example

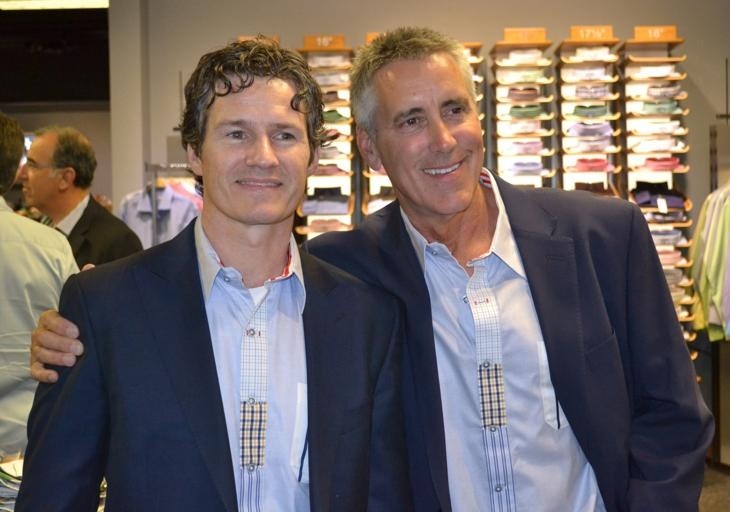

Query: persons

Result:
[0,109,95,454]
[16,122,145,271]
[27,26,720,512]
[14,36,409,512]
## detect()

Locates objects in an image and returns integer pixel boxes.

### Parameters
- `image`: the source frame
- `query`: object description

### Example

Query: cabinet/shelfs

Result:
[120,26,702,387]
